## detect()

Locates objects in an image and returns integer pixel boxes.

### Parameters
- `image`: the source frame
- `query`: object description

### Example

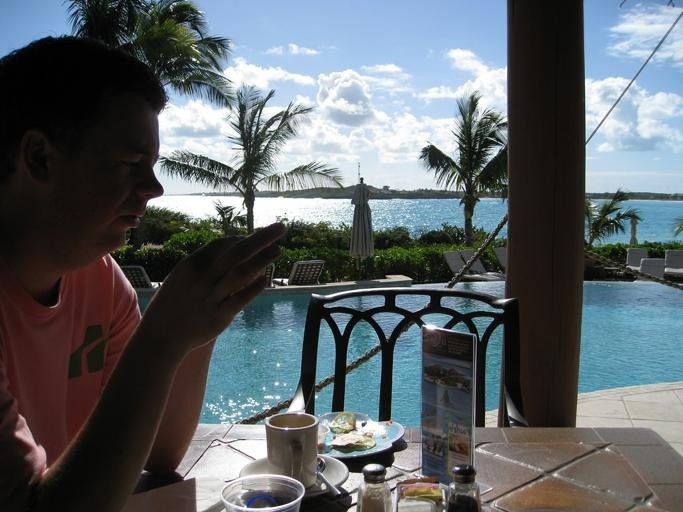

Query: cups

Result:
[266,412,316,487]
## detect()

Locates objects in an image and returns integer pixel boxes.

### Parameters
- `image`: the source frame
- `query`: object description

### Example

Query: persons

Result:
[0,35,288,512]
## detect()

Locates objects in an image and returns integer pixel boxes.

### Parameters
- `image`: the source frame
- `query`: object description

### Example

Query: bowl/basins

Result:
[220,474,303,512]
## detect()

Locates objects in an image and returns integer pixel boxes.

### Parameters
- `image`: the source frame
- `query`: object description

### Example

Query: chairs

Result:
[264,260,323,287]
[118,265,157,288]
[625,245,683,279]
[443,247,506,281]
[286,289,528,427]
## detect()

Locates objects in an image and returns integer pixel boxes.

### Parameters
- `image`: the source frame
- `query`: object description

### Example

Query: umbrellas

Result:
[350,177,373,280]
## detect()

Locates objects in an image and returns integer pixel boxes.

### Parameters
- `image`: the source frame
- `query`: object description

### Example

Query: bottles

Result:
[358,463,390,510]
[443,466,480,511]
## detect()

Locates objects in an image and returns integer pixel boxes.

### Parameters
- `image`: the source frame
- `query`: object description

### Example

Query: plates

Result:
[305,415,406,458]
[240,455,346,494]
